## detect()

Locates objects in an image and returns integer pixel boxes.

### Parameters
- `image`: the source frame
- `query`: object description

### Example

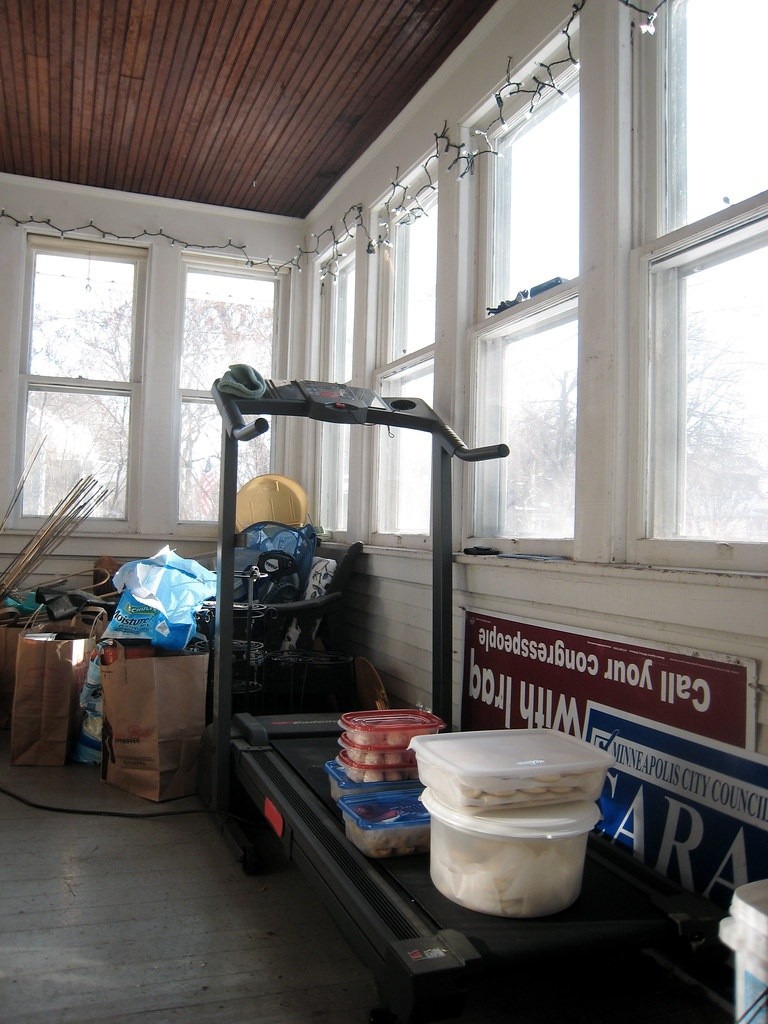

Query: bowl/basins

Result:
[418,787,602,917]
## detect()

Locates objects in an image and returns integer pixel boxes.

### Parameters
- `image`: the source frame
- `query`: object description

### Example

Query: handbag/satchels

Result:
[98,631,210,802]
[0,602,104,767]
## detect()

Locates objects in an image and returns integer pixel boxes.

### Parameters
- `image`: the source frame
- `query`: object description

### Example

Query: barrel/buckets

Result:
[717,879,768,1024]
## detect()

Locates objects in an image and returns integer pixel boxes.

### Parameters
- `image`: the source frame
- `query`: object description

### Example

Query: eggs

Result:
[344,730,430,782]
